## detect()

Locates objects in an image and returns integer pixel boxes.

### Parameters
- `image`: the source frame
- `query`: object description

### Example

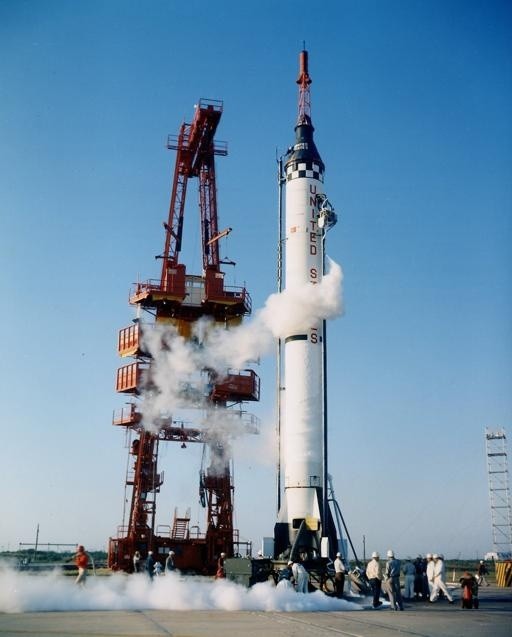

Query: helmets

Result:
[288,561,293,565]
[372,552,379,558]
[426,554,444,559]
[336,552,342,556]
[387,551,393,557]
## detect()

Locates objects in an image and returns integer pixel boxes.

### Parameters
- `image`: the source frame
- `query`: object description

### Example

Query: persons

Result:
[385,550,404,611]
[414,553,456,604]
[72,544,90,590]
[146,550,154,578]
[402,556,415,599]
[288,561,309,594]
[334,552,345,599]
[477,561,489,587]
[165,551,176,575]
[366,551,382,609]
[217,552,227,579]
[133,550,142,574]
